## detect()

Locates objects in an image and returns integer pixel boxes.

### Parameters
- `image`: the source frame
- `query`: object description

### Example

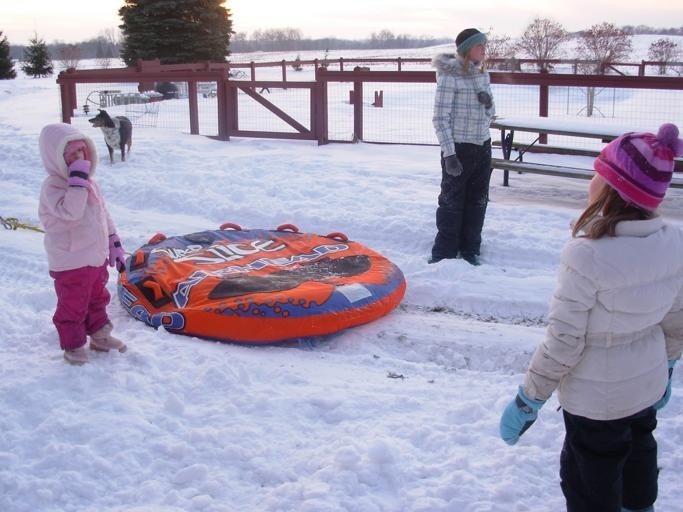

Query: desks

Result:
[490,118,683,187]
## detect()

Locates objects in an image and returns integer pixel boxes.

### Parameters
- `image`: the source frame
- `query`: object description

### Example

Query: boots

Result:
[89,322,127,353]
[63,345,89,367]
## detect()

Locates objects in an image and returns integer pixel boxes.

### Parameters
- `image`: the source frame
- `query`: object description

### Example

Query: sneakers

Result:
[462,253,482,265]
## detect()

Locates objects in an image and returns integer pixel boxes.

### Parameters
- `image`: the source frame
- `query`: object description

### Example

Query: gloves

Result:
[499,385,546,445]
[69,159,91,188]
[477,91,492,109]
[652,360,676,410]
[109,234,126,273]
[444,155,463,176]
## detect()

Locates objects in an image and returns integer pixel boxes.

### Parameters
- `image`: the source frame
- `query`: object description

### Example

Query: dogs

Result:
[88,107,133,164]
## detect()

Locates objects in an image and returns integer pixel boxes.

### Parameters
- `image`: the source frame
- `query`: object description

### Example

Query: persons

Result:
[499,123,683,512]
[38,121,128,366]
[427,29,496,265]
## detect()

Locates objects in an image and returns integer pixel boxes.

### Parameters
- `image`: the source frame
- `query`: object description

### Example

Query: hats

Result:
[594,124,683,212]
[456,28,487,53]
[63,140,87,160]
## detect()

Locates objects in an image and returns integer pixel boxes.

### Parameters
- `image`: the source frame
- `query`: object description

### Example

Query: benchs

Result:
[489,157,683,189]
[490,140,683,174]
